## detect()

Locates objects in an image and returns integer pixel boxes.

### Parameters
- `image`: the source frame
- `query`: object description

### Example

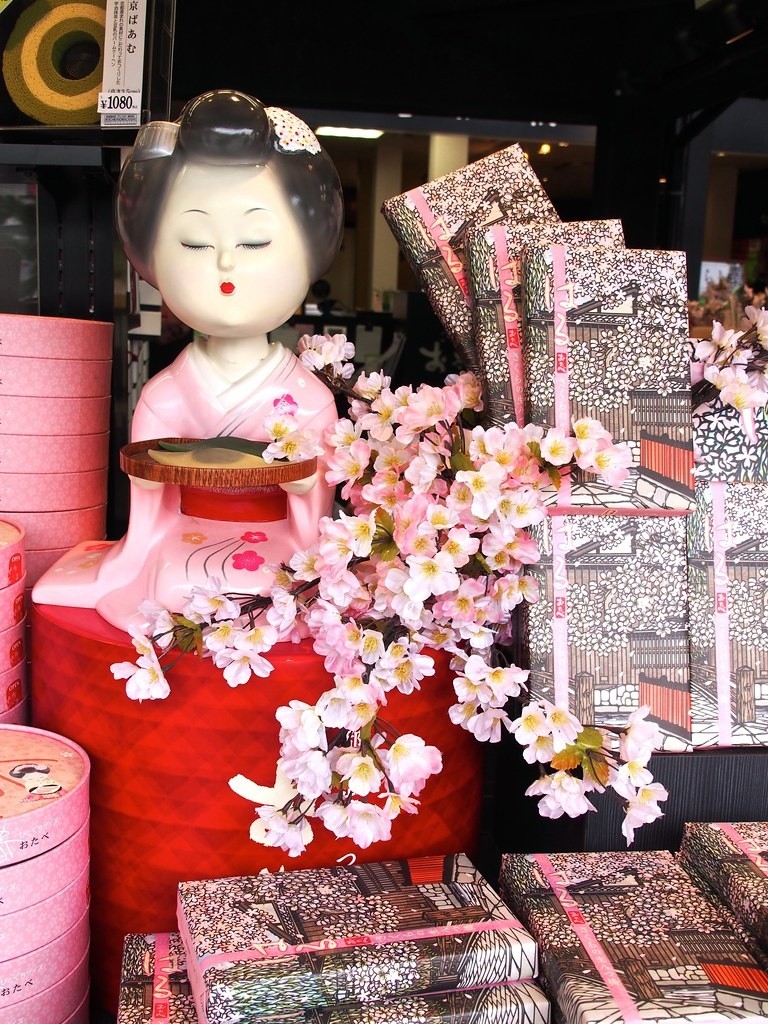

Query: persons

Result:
[30,92,343,641]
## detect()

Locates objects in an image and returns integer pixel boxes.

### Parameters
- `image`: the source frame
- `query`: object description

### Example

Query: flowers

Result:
[691,303,768,422]
[107,332,671,860]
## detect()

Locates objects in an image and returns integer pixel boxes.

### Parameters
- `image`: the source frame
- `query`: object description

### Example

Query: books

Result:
[379,142,768,753]
[118,822,768,1024]
